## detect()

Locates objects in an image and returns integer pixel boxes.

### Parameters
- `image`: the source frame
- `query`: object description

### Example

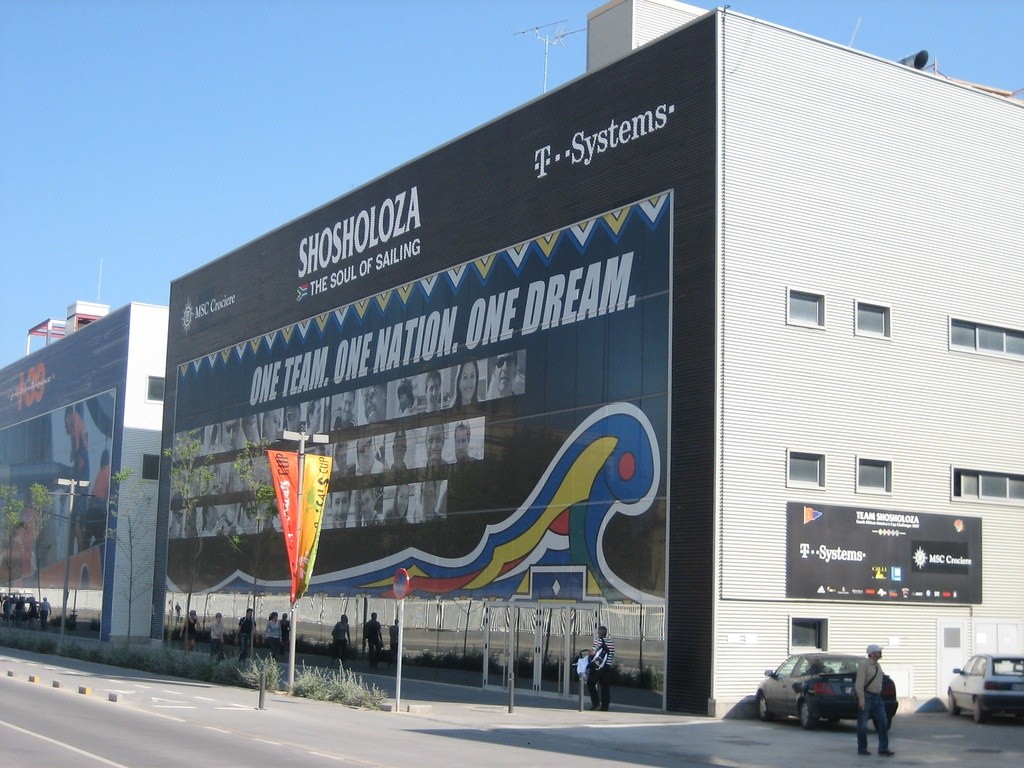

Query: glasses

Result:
[216,615,221,617]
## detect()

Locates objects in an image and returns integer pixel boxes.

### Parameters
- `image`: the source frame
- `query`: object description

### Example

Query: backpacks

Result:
[590,637,609,670]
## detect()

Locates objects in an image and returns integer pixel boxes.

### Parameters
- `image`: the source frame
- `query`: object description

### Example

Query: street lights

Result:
[54,477,92,654]
[274,430,330,697]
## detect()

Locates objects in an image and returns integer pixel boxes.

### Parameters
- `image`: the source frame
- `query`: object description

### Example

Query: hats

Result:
[867,645,884,655]
[43,597,47,600]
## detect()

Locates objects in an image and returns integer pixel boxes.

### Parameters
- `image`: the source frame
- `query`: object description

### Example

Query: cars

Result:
[756,652,899,731]
[946,653,1024,724]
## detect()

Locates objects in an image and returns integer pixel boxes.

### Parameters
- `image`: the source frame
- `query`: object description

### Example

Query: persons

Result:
[234,608,259,663]
[0,405,110,586]
[168,351,518,539]
[330,615,351,669]
[179,610,202,655]
[3,596,51,631]
[365,613,399,669]
[855,644,895,756]
[262,612,291,663]
[209,613,225,663]
[587,626,615,711]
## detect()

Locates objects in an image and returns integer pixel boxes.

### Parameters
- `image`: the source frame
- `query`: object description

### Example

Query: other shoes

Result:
[598,705,609,711]
[858,749,871,755]
[589,703,600,710]
[878,749,895,756]
